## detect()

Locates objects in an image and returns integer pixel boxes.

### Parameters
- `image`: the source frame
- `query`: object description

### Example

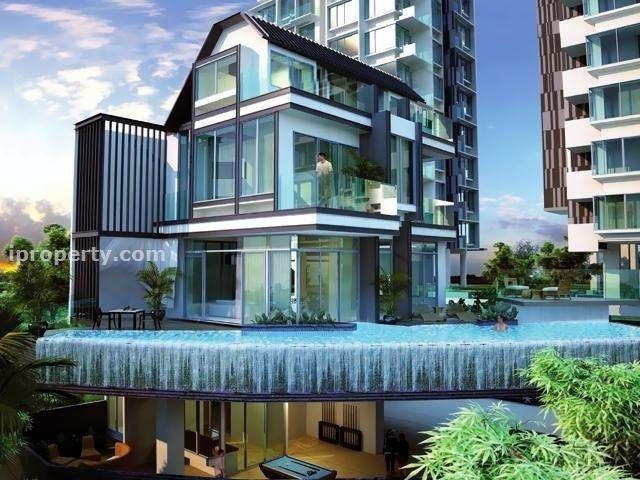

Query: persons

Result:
[316,153,333,208]
[214,449,225,480]
[494,314,508,330]
[384,432,397,472]
[396,432,411,469]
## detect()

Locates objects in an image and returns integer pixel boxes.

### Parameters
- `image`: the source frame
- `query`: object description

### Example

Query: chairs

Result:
[503,277,577,300]
[46,443,75,466]
[91,303,117,329]
[78,435,101,460]
[138,303,164,330]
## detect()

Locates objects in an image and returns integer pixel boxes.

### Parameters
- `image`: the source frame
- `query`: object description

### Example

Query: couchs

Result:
[80,441,135,469]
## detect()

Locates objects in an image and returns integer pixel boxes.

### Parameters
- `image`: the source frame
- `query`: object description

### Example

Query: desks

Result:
[107,307,147,329]
[258,454,338,480]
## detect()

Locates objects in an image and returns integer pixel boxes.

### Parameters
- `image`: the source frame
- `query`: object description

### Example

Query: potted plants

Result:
[136,260,181,323]
[12,282,58,339]
[375,265,410,325]
